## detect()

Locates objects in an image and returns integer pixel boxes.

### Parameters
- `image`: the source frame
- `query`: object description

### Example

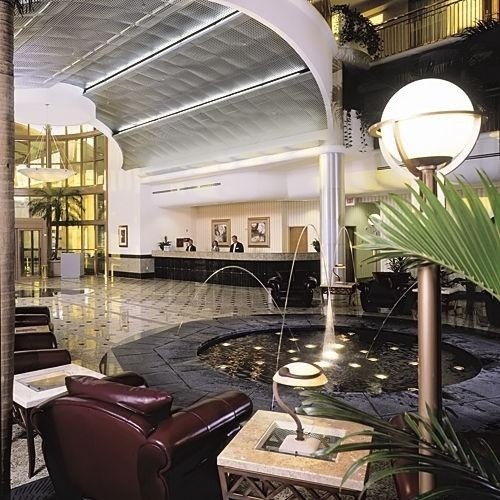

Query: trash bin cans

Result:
[61,252,84,278]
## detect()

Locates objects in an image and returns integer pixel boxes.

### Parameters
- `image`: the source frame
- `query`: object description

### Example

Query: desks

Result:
[15,326,55,332]
[14,361,109,478]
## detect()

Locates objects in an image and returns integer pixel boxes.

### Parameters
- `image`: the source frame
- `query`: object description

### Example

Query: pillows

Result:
[63,372,172,424]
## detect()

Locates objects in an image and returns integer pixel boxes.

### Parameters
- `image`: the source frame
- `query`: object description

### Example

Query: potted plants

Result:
[157,236,172,251]
[331,3,385,61]
[27,184,86,277]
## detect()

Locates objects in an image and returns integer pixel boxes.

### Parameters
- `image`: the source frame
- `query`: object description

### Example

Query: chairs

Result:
[14,307,52,327]
[388,415,500,500]
[268,271,317,307]
[357,272,418,315]
[14,332,71,374]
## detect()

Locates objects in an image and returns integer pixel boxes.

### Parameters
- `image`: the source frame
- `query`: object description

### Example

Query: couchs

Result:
[30,372,252,499]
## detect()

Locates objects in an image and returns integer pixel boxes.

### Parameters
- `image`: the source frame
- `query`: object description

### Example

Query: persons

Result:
[186,239,196,251]
[211,241,219,252]
[230,235,244,252]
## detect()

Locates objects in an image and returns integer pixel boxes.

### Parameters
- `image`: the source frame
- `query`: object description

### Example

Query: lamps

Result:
[13,83,96,183]
[272,361,329,457]
[333,264,346,283]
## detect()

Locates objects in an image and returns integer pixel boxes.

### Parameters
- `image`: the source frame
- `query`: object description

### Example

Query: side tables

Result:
[319,282,356,306]
[411,287,460,317]
[217,410,374,500]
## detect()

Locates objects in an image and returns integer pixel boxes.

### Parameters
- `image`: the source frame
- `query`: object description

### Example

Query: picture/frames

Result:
[211,219,231,247]
[118,225,128,247]
[247,217,270,248]
